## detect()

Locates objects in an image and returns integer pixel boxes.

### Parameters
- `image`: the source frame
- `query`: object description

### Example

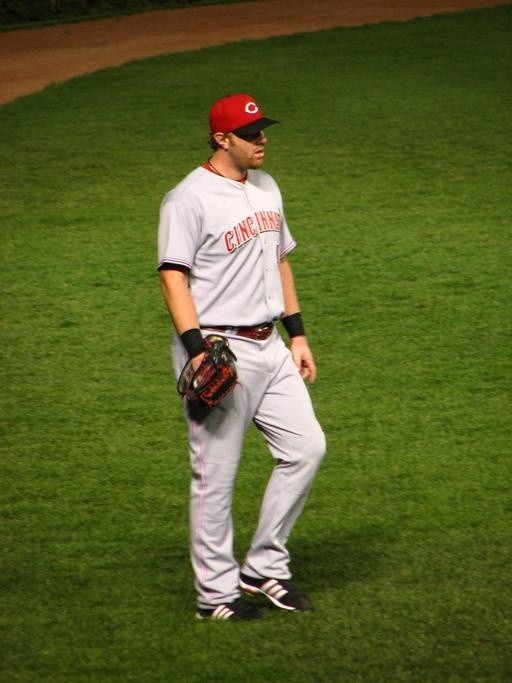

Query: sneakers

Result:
[238,572,313,614]
[194,596,265,621]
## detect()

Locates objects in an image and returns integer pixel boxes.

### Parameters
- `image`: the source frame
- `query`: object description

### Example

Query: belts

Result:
[204,322,273,342]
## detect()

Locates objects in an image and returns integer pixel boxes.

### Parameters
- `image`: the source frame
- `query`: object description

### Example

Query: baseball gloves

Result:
[177,334,239,423]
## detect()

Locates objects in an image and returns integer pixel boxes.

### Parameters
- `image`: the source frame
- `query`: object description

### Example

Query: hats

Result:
[208,93,281,138]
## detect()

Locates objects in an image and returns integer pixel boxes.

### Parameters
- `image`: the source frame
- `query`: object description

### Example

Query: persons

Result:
[153,92,329,624]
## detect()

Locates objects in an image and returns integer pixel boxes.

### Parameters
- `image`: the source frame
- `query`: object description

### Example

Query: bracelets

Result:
[281,311,307,338]
